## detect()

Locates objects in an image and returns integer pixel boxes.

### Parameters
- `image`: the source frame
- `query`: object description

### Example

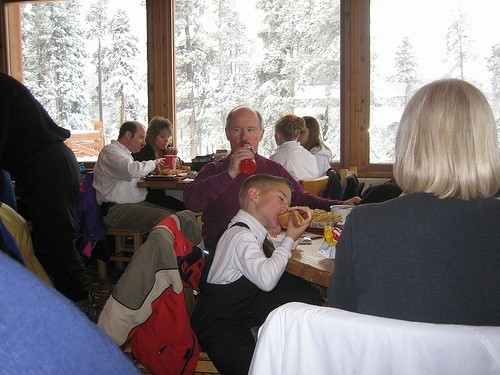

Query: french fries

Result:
[310,210,343,222]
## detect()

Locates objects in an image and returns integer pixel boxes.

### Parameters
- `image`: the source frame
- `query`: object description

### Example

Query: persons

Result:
[329,78,499,327]
[93,121,182,231]
[267,114,320,182]
[297,116,332,179]
[189,174,312,375]
[131,116,186,212]
[183,104,363,306]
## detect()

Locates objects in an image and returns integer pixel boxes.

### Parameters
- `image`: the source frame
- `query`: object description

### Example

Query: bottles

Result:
[237,140,257,176]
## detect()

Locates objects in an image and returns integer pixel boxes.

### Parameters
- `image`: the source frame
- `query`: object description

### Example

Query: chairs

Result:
[147,210,221,375]
[85,171,149,280]
[247,302,500,375]
[297,166,358,197]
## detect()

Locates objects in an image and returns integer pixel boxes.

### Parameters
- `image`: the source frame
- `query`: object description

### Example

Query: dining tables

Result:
[263,228,335,287]
[136,171,199,190]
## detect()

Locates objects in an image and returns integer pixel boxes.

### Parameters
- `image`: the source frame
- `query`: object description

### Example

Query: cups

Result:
[164,154,178,169]
[330,205,352,225]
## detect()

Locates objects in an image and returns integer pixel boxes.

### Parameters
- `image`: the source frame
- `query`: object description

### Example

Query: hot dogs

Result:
[278,205,312,228]
